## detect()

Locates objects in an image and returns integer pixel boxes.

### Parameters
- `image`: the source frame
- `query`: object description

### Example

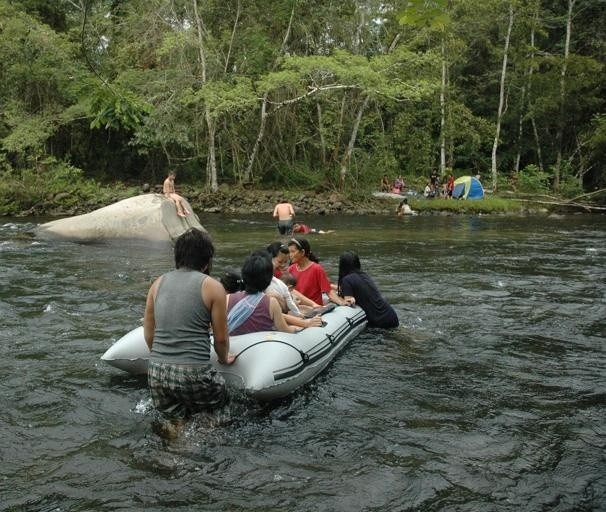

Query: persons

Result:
[395,199,413,216]
[163,171,192,217]
[381,174,390,193]
[394,175,405,191]
[219,236,399,334]
[423,168,454,201]
[143,228,236,419]
[273,200,337,237]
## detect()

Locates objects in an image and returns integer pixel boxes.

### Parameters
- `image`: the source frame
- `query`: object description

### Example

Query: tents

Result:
[452,176,484,200]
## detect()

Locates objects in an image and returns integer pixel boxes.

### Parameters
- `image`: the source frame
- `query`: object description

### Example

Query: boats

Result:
[99,287,370,402]
